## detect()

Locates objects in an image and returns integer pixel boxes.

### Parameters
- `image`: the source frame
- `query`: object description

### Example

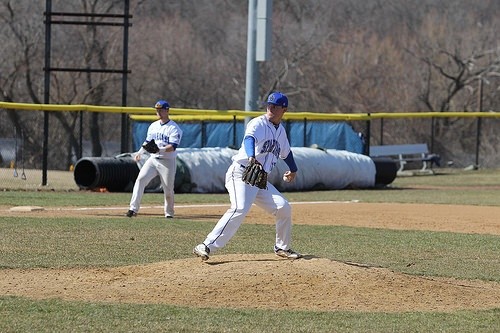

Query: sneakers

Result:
[165,216,174,218]
[124,210,136,217]
[194,243,210,261]
[274,244,301,259]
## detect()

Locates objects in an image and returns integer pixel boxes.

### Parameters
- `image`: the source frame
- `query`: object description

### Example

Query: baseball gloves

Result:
[142,138,160,155]
[241,159,267,190]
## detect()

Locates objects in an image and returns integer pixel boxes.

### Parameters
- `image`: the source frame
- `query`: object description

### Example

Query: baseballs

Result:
[282,175,292,183]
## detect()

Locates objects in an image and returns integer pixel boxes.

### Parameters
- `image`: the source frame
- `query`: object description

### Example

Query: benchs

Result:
[369,143,430,173]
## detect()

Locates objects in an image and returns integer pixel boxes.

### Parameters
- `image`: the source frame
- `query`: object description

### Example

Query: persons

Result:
[194,92,302,260]
[127,99,182,218]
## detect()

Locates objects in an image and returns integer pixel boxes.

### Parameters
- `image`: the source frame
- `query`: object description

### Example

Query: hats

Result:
[155,100,170,109]
[262,92,288,107]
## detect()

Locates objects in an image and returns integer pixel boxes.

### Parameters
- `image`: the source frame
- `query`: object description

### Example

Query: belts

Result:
[241,164,245,168]
[155,157,164,160]
